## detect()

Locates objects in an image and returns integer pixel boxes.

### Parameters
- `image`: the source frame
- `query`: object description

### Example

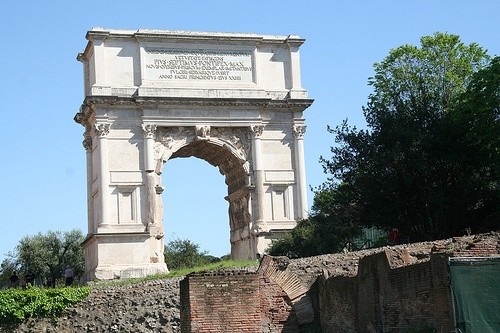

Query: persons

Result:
[10,272,18,288]
[390,229,399,242]
[64,265,74,285]
